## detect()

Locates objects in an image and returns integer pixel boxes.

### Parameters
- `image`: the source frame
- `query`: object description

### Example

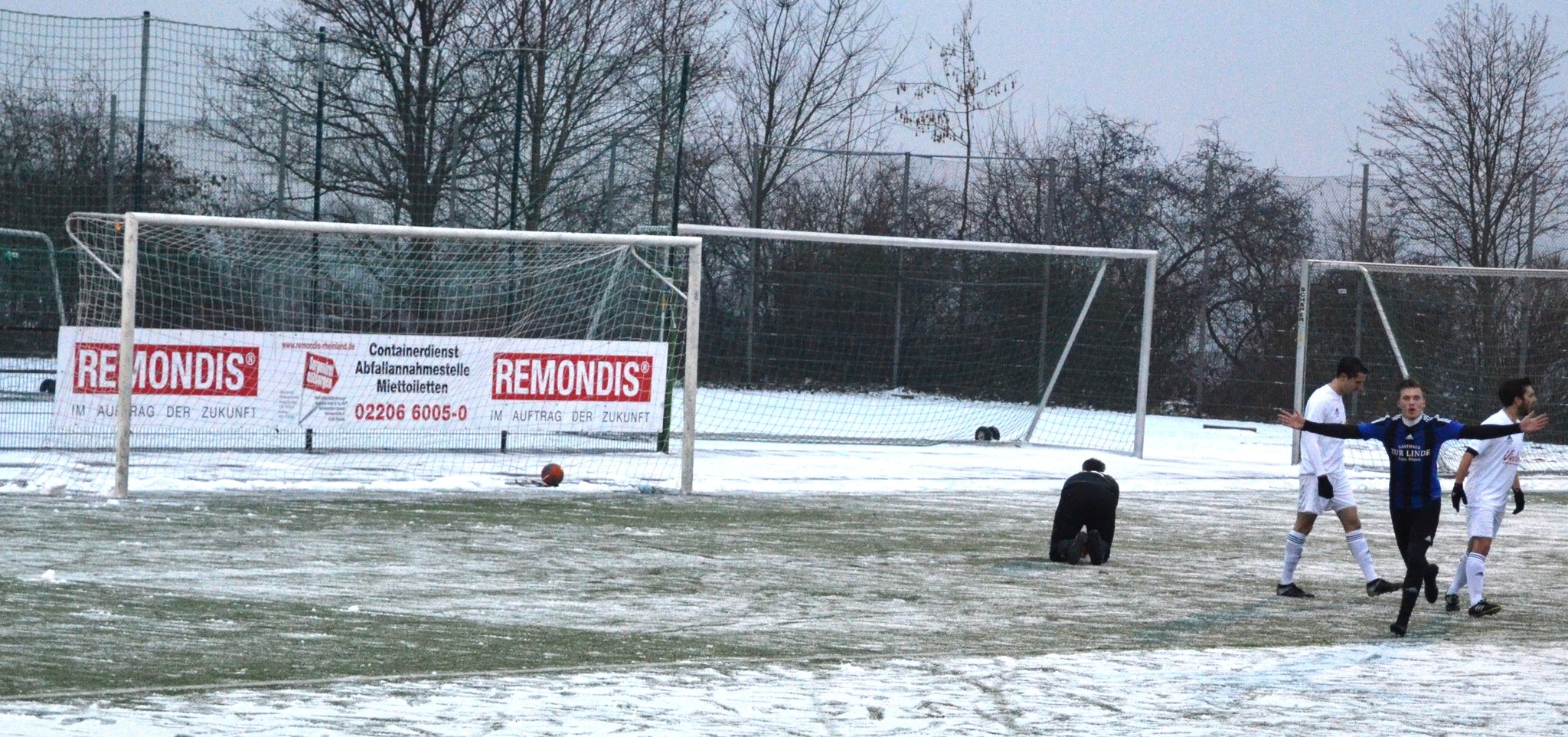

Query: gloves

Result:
[1318,476,1335,500]
[1511,490,1525,515]
[1451,482,1467,512]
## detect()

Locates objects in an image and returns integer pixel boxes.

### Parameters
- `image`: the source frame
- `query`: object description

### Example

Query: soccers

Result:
[541,463,564,485]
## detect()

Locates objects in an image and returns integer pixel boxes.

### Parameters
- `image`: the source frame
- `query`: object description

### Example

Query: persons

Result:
[1444,378,1537,616]
[1277,377,1548,634]
[1049,458,1119,566]
[1276,356,1402,598]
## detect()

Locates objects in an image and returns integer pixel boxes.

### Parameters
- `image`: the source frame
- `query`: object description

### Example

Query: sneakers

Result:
[1389,622,1407,637]
[1468,600,1501,617]
[1366,577,1404,597]
[1424,563,1440,604]
[1278,582,1314,599]
[1066,531,1086,566]
[1087,529,1104,566]
[1444,592,1461,612]
